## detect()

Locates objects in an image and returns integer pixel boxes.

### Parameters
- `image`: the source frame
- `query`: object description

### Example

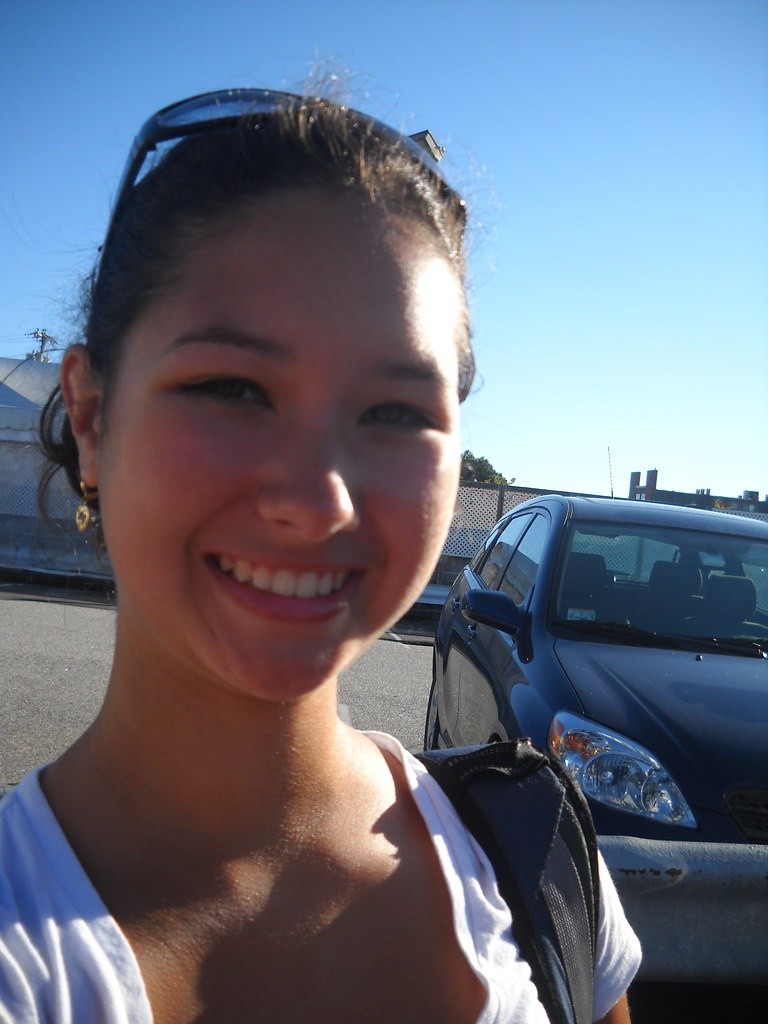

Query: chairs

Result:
[650,560,703,599]
[689,572,758,637]
[564,553,629,623]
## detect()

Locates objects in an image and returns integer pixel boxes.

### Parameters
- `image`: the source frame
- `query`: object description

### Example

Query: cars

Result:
[423,494,768,843]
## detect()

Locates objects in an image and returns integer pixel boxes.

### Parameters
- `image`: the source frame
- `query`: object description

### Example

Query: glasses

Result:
[91,89,468,308]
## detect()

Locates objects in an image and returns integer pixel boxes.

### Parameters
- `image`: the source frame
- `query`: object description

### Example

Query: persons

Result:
[0,88,646,1024]
